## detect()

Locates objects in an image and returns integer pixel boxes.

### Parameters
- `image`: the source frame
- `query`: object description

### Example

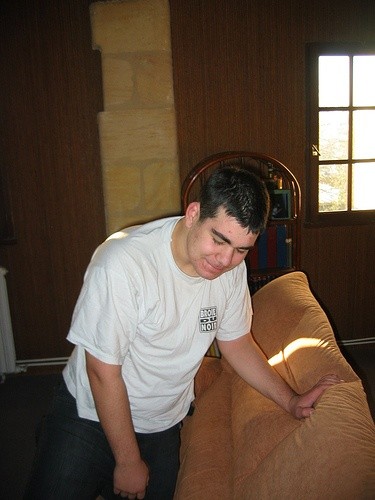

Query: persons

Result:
[25,166,346,500]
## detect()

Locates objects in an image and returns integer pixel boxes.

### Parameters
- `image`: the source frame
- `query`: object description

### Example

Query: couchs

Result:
[173,270,375,500]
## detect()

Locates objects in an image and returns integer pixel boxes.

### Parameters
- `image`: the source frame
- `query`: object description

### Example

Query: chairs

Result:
[182,151,301,295]
